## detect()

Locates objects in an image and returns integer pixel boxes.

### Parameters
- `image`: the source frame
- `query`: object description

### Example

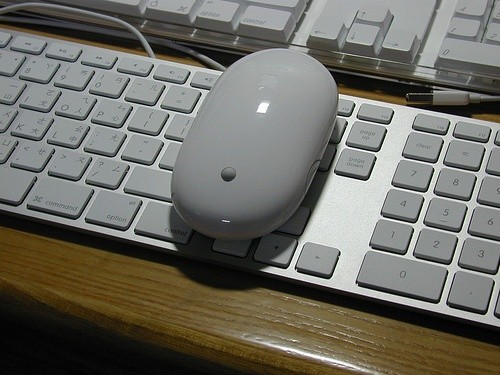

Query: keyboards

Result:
[0,24,496,331]
[0,1,496,96]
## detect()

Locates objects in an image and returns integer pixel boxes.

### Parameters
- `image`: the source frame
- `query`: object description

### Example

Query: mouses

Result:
[170,48,339,229]
